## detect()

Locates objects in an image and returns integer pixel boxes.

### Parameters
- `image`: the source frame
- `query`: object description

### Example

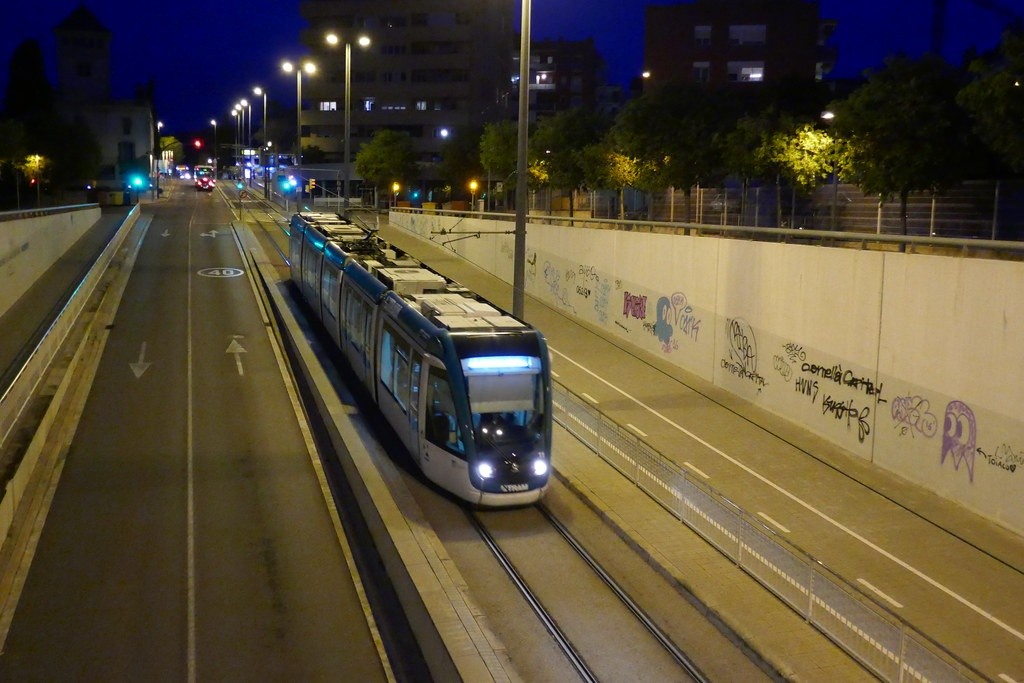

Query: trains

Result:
[288,210,553,508]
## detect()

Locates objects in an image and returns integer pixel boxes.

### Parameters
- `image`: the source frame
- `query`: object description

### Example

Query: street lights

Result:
[822,111,839,231]
[155,121,164,199]
[231,109,241,147]
[325,31,372,214]
[283,59,317,211]
[469,179,479,211]
[211,120,217,156]
[254,86,267,148]
[236,104,244,144]
[241,99,252,163]
[392,182,400,211]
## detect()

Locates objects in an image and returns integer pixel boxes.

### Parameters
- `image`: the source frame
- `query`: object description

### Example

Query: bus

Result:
[194,165,216,192]
